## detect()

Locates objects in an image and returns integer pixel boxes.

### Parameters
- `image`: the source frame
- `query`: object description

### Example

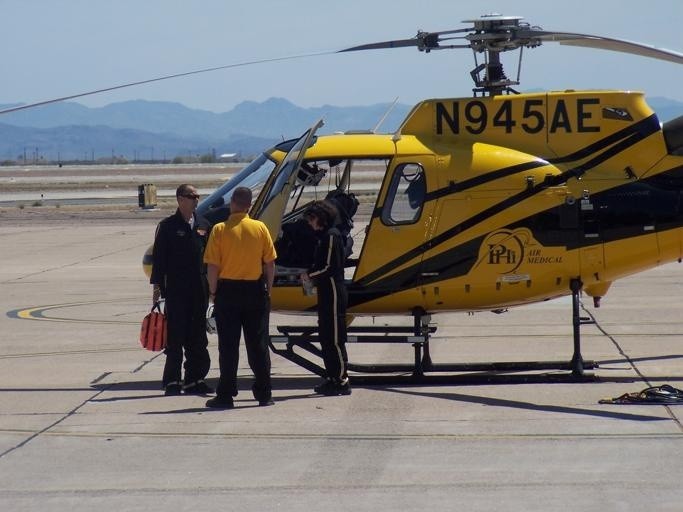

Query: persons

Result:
[150,184,215,395]
[203,187,278,408]
[403,180,425,221]
[300,201,351,395]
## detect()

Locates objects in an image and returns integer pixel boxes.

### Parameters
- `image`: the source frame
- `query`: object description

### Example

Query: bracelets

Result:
[209,292,217,296]
[153,288,160,294]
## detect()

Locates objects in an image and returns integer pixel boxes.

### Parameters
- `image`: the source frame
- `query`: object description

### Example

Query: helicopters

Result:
[1,10,682,387]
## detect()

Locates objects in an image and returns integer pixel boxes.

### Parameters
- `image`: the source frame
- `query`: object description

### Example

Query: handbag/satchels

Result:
[140,303,168,352]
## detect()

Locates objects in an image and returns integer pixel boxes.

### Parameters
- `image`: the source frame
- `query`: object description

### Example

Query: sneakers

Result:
[313,378,352,396]
[165,381,215,396]
[258,395,276,407]
[205,394,235,409]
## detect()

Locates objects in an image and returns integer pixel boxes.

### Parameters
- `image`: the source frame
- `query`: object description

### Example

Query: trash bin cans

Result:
[138,184,157,209]
